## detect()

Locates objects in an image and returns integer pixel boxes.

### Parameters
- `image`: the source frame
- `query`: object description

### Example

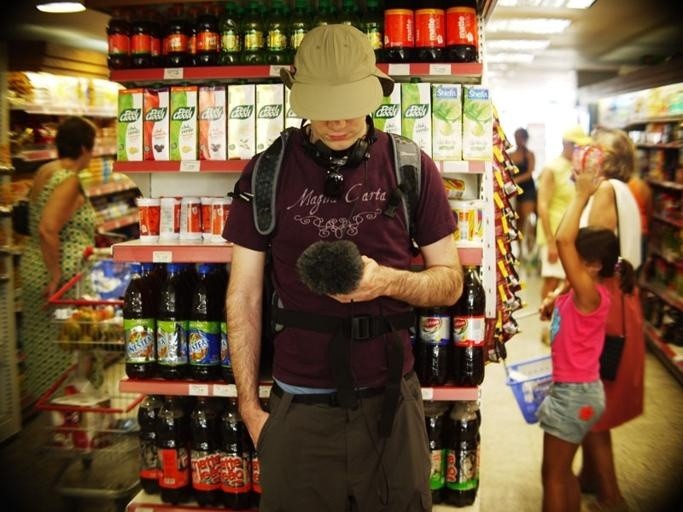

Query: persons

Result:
[507,122,653,349]
[530,161,634,509]
[538,129,644,511]
[218,22,464,509]
[15,113,109,448]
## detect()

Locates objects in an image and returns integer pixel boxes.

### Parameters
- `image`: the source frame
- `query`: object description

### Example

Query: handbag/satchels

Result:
[597,332,625,380]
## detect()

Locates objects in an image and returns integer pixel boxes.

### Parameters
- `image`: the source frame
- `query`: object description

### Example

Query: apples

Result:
[58,305,114,350]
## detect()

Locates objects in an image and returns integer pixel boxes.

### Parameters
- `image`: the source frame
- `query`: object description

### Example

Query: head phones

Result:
[300,116,376,167]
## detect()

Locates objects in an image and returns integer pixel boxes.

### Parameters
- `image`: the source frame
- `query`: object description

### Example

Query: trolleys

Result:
[90,230,132,249]
[38,244,147,511]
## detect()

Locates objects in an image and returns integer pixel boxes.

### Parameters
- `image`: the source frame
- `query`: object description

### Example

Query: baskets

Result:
[501,306,553,424]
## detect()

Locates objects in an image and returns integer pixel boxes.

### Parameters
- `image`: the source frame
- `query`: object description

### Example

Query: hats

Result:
[289,25,395,123]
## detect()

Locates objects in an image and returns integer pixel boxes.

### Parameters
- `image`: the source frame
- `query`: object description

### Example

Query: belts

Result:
[271,371,414,406]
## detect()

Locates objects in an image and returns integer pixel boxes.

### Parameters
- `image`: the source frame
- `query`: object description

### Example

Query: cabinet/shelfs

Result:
[109,4,496,512]
[590,113,683,391]
[0,69,141,441]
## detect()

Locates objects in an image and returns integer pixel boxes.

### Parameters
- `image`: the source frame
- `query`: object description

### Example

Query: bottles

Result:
[450,267,486,387]
[136,393,264,505]
[447,401,480,508]
[108,1,480,67]
[423,401,450,506]
[121,263,273,380]
[418,305,451,386]
